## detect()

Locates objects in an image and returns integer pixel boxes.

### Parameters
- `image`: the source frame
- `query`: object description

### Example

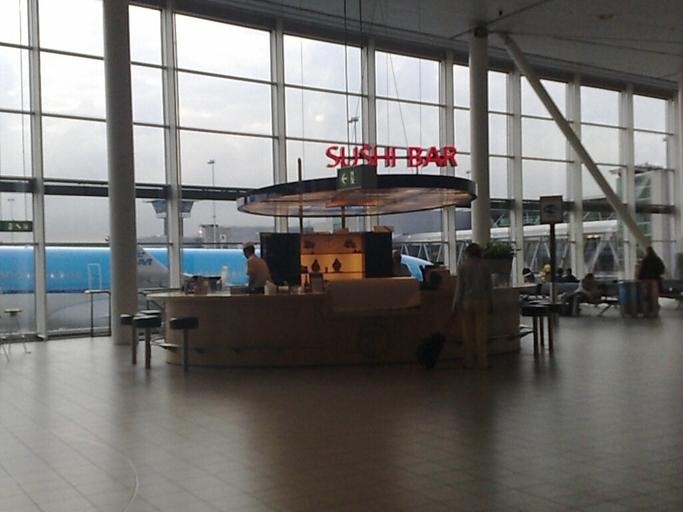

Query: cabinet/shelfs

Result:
[300,251,364,274]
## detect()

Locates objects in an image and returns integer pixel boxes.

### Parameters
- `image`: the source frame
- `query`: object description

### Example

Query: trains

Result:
[0,245,438,343]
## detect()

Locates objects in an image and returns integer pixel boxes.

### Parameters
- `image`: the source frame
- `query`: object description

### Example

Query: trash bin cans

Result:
[616,279,640,319]
[639,279,659,318]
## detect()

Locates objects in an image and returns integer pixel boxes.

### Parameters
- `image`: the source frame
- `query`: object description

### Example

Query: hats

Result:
[238,239,255,248]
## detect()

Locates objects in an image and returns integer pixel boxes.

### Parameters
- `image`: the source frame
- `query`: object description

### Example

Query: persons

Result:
[523,264,578,295]
[639,247,665,319]
[392,250,411,276]
[562,274,599,318]
[451,243,494,371]
[244,246,270,293]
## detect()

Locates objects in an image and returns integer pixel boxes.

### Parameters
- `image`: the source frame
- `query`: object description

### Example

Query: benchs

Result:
[539,280,683,316]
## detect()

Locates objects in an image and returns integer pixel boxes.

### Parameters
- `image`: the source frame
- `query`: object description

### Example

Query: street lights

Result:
[207,159,218,248]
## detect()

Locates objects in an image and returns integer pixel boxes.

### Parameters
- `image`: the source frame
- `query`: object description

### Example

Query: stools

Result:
[521,299,563,356]
[120,308,199,373]
